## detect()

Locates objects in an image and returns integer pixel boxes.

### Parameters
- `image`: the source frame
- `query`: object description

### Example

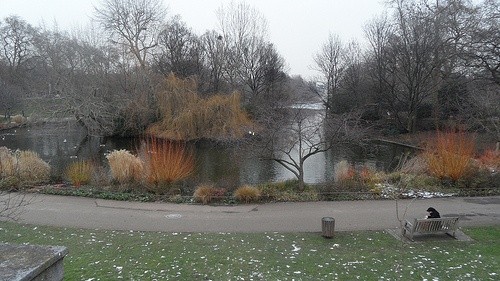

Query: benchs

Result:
[402,218,459,241]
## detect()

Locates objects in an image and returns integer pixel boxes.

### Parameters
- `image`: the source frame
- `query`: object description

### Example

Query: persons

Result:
[425,207,440,219]
